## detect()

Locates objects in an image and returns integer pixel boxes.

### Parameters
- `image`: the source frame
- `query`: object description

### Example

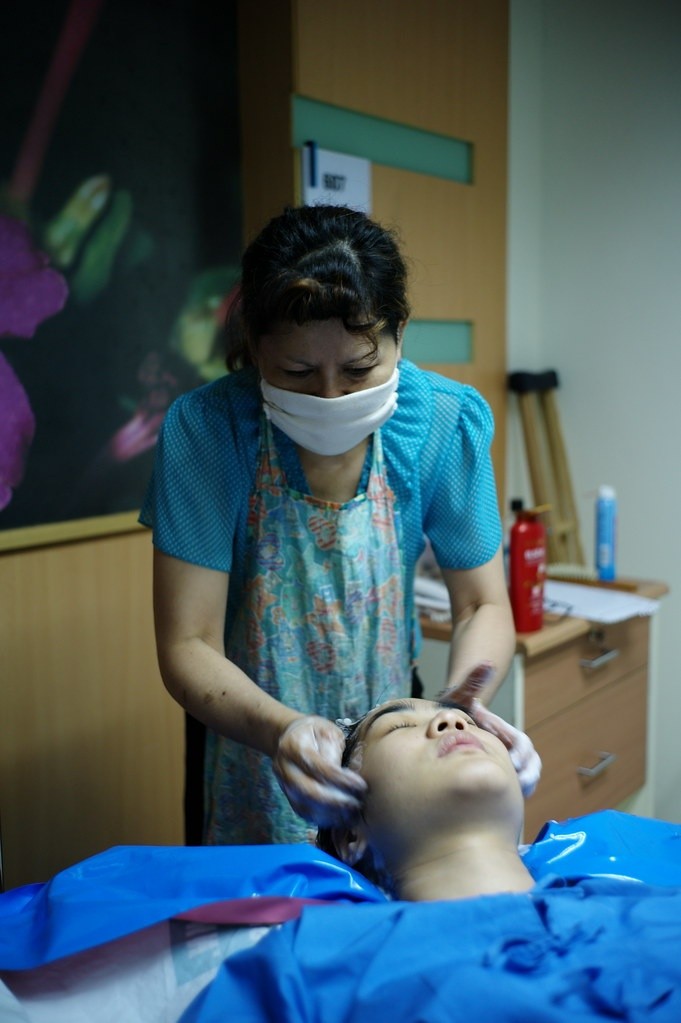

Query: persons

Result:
[175,695,681,1023]
[152,203,545,847]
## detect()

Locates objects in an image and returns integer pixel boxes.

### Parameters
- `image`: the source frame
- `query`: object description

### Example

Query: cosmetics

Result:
[593,485,618,584]
[507,489,554,634]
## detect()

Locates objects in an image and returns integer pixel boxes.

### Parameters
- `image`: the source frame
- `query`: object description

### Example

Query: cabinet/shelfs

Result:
[419,574,668,842]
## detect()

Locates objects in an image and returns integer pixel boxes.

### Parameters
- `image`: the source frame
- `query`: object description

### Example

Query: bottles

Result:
[595,486,615,585]
[511,509,546,631]
